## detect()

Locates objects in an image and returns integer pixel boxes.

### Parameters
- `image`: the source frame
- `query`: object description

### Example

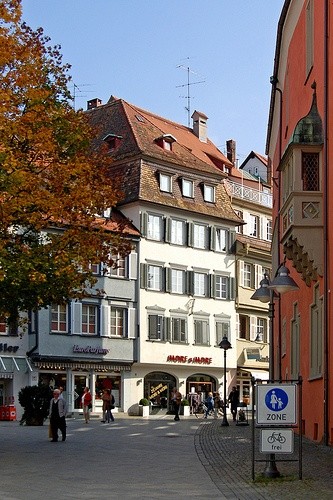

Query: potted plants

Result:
[139,398,149,416]
[181,400,190,416]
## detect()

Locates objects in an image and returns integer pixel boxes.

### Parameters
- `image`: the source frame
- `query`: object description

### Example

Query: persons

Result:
[82,387,92,424]
[204,391,224,419]
[101,388,115,423]
[228,386,240,421]
[171,387,182,420]
[49,386,67,442]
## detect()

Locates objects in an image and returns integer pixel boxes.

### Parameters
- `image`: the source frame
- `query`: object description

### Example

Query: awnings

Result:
[240,368,270,380]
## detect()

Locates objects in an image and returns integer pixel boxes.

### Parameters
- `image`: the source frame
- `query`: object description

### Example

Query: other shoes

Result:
[62,436,66,441]
[50,439,58,442]
[101,421,105,423]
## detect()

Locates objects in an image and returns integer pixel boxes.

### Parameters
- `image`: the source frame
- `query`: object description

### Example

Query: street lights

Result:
[217,333,232,427]
[251,256,302,478]
